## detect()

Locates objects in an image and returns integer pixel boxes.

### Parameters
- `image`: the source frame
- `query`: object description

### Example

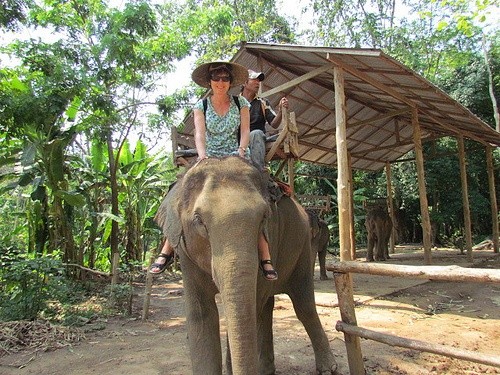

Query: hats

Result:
[190,60,249,87]
[247,69,264,81]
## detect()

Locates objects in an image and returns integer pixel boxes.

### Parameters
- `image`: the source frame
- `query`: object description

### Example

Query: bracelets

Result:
[239,146,246,152]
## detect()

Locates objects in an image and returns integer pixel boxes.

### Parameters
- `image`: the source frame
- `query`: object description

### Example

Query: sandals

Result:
[259,259,278,281]
[149,253,173,274]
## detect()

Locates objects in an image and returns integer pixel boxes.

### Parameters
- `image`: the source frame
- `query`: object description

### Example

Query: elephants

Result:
[152,155,341,375]
[365,209,393,262]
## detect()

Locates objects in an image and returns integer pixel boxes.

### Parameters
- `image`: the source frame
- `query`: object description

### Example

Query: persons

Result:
[237,69,289,173]
[149,60,278,281]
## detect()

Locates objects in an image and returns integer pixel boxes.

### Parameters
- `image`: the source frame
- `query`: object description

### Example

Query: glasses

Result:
[210,75,230,83]
